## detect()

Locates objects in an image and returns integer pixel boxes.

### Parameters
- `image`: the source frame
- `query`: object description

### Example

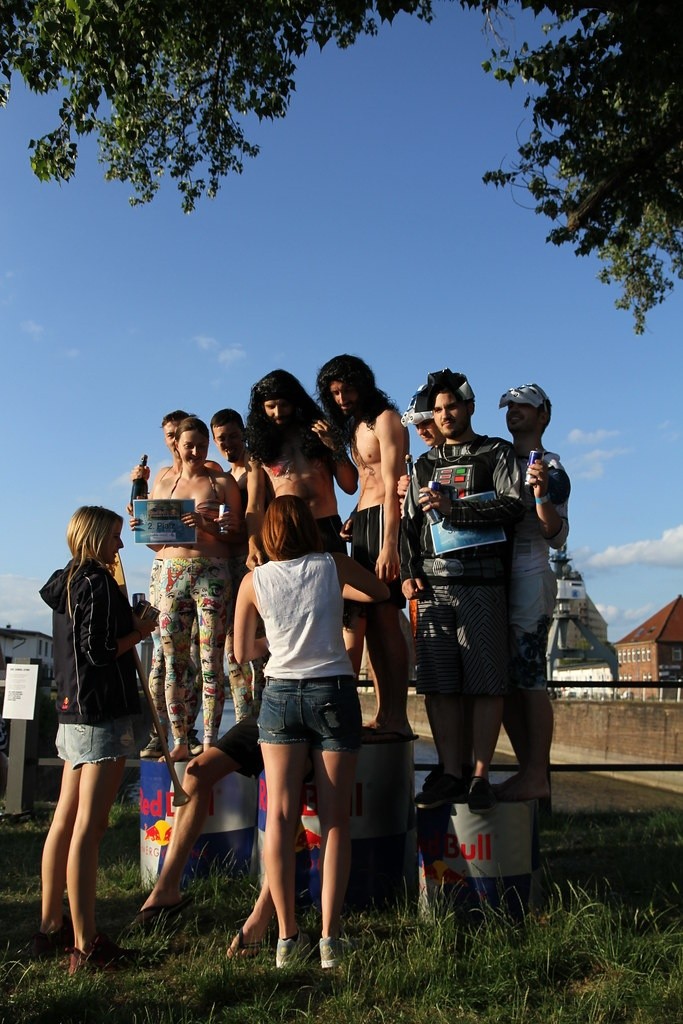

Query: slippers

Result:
[226,926,262,961]
[126,893,194,935]
[361,725,419,744]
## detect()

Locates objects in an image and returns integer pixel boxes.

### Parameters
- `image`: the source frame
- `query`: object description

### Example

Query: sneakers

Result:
[140,731,168,757]
[69,933,138,977]
[276,927,311,969]
[31,913,75,958]
[188,729,203,755]
[319,937,356,969]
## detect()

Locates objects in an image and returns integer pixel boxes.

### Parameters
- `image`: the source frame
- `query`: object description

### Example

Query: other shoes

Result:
[463,758,475,784]
[469,776,497,814]
[414,774,466,809]
[422,762,444,791]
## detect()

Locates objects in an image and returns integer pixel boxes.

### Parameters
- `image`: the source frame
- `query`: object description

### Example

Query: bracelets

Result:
[534,491,551,504]
[441,503,452,517]
[132,629,143,644]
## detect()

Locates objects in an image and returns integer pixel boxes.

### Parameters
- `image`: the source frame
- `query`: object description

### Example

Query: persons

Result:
[126,406,272,764]
[233,493,392,969]
[122,601,369,961]
[397,369,527,814]
[31,505,160,978]
[491,384,573,807]
[317,354,413,744]
[244,369,360,628]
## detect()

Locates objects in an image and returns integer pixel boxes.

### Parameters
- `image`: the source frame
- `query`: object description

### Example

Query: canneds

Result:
[218,504,231,534]
[524,448,542,486]
[419,480,442,526]
[132,593,161,622]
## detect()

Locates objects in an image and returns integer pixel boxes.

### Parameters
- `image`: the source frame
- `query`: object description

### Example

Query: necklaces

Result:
[442,435,476,463]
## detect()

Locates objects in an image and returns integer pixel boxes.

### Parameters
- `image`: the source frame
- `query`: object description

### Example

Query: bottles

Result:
[129,455,149,509]
[404,454,414,476]
